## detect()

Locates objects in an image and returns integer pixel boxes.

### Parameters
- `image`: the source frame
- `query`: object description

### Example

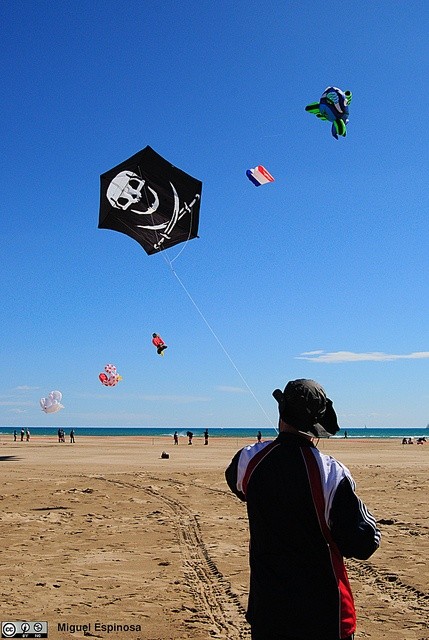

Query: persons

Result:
[26,428,31,441]
[60,430,65,442]
[14,430,17,441]
[257,431,262,443]
[225,378,381,639]
[401,437,427,445]
[187,431,193,445]
[70,429,75,443]
[58,428,65,442]
[203,429,208,445]
[21,428,24,441]
[174,431,178,445]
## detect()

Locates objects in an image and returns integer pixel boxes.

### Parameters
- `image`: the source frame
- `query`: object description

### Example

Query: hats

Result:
[272,378,340,438]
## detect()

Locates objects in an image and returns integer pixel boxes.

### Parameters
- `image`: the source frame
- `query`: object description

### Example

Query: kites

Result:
[98,145,202,256]
[305,86,352,140]
[40,391,64,414]
[98,364,122,387]
[246,165,275,187]
[152,333,167,356]
